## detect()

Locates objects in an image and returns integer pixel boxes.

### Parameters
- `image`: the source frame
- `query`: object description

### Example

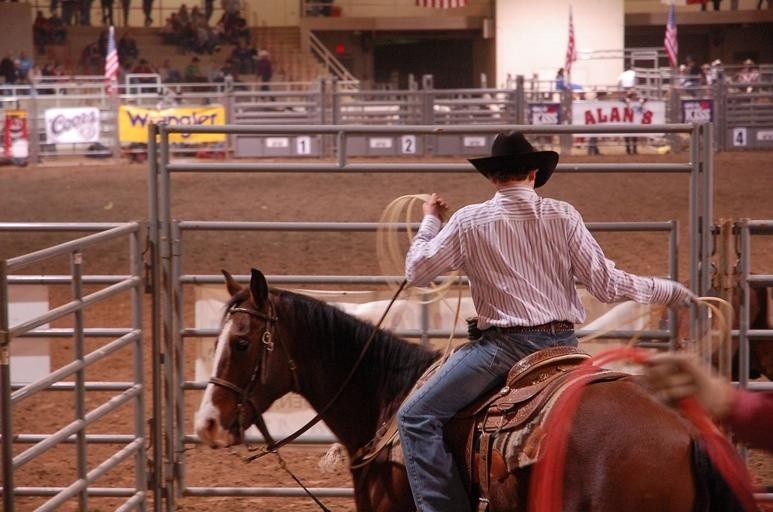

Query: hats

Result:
[466,130,560,188]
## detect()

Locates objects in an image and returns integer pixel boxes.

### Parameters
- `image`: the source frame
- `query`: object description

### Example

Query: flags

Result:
[662,2,680,70]
[565,7,577,80]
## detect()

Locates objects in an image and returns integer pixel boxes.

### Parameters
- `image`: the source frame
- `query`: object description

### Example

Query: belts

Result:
[503,322,575,333]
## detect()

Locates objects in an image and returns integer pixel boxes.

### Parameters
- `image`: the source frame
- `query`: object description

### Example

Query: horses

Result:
[191,265,754,512]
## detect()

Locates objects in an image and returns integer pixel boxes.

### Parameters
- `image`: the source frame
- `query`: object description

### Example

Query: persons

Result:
[394,128,707,512]
[699,1,772,13]
[676,51,762,103]
[618,61,638,100]
[328,46,351,79]
[553,67,584,126]
[620,87,644,156]
[584,87,607,156]
[0,0,278,161]
